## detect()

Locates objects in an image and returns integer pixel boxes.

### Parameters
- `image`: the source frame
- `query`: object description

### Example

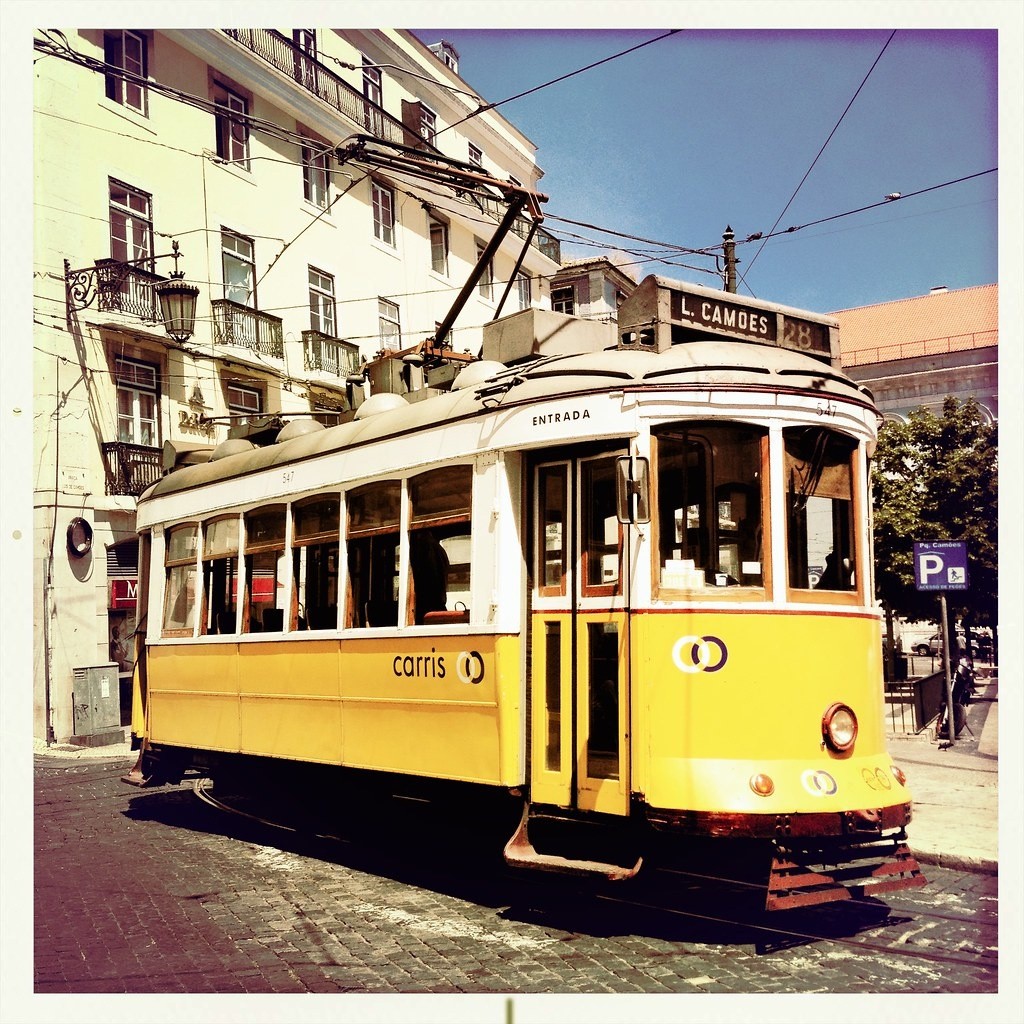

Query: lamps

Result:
[63,238,202,346]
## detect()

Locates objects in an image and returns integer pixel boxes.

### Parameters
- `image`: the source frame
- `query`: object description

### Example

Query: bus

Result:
[122,273,929,912]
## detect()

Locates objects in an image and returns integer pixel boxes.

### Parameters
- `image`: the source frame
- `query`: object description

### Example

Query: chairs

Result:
[306,605,337,629]
[216,612,237,634]
[424,610,470,625]
[364,600,399,627]
[261,607,283,632]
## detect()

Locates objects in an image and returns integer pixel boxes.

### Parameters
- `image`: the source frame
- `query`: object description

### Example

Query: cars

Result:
[910,630,981,659]
